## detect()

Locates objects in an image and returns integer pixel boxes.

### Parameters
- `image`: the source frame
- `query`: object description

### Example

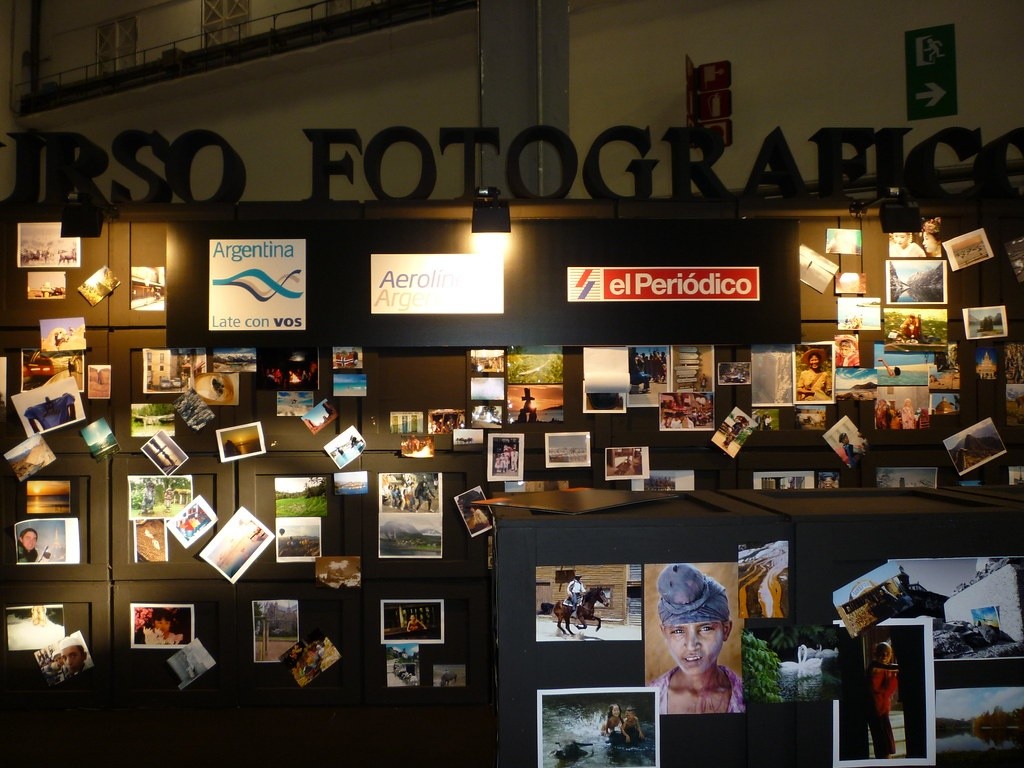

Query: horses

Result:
[541,585,609,635]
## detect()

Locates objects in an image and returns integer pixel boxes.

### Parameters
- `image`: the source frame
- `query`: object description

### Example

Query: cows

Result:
[551,739,594,761]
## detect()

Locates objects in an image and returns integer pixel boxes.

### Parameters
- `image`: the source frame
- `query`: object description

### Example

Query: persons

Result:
[337,435,359,454]
[22,246,75,296]
[17,528,39,563]
[395,216,1024,759]
[353,351,359,364]
[40,637,90,681]
[151,608,183,645]
[185,515,195,537]
[225,440,241,456]
[289,537,303,547]
[139,483,172,513]
[57,326,75,342]
[264,367,314,386]
[303,645,324,681]
[155,290,160,302]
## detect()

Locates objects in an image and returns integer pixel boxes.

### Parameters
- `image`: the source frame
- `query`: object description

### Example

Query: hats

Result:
[802,348,827,367]
[52,651,61,659]
[839,433,846,443]
[60,637,83,652]
